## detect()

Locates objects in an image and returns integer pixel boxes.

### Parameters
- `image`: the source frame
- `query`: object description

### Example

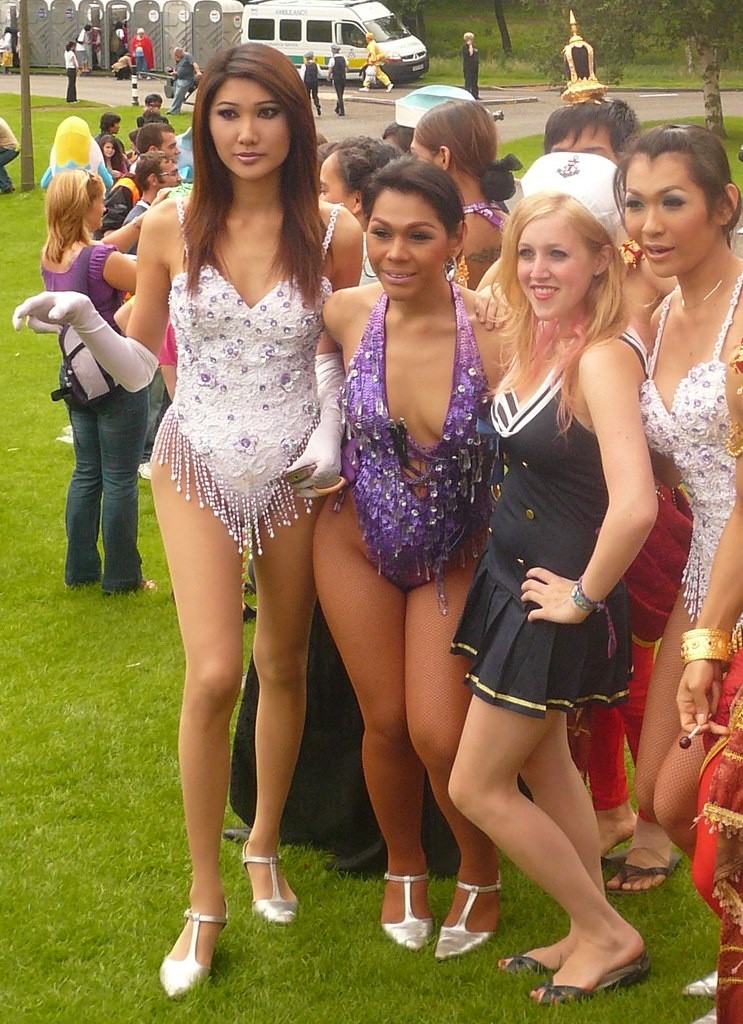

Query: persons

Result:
[312,153,522,964]
[326,44,350,116]
[75,24,102,71]
[39,84,743,896]
[675,337,743,1024]
[0,27,19,75]
[448,150,657,1011]
[64,41,80,104]
[613,125,743,1024]
[462,32,482,100]
[0,116,21,195]
[110,21,154,81]
[13,41,364,1007]
[300,50,323,116]
[358,32,393,93]
[165,47,203,115]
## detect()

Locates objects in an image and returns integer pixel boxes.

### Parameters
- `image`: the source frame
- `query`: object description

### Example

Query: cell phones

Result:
[286,464,317,483]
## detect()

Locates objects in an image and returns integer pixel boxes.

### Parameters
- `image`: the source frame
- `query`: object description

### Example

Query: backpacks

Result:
[57,248,122,408]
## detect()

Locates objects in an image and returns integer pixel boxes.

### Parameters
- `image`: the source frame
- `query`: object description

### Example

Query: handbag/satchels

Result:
[1,52,13,67]
[365,65,377,84]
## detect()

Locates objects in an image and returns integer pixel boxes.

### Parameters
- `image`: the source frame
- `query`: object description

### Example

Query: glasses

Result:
[158,169,177,177]
[76,167,94,187]
[148,104,162,109]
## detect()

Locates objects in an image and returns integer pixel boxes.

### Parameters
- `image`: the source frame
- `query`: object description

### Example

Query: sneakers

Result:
[139,462,151,480]
[359,87,369,91]
[386,84,393,93]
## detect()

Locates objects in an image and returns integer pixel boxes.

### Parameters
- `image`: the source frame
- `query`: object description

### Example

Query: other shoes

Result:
[682,970,717,998]
[0,185,15,194]
[691,1007,715,1024]
[102,580,156,592]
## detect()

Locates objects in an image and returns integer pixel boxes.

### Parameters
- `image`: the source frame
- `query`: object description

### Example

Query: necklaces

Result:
[680,276,725,310]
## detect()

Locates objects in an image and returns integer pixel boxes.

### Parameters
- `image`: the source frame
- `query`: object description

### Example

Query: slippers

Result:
[499,947,561,976]
[532,948,651,1006]
[601,851,681,893]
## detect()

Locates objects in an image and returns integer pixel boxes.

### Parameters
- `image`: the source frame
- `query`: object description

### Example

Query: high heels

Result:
[381,872,435,950]
[159,897,229,999]
[242,839,299,924]
[435,871,501,961]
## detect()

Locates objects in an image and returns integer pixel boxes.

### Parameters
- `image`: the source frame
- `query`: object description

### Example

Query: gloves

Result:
[11,289,159,392]
[284,352,348,499]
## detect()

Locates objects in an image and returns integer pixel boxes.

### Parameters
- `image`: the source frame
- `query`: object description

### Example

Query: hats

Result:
[520,153,627,250]
[366,33,374,39]
[331,44,341,52]
[304,51,314,58]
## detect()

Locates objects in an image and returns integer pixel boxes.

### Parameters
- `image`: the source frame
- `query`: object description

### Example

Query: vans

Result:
[241,0,429,89]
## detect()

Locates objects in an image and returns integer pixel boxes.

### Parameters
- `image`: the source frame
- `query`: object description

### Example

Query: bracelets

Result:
[678,627,734,672]
[577,574,617,660]
[571,580,594,612]
